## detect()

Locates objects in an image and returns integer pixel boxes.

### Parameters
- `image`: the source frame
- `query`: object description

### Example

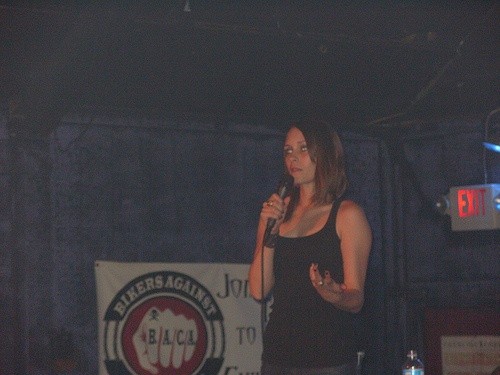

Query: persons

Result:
[249,117,371,375]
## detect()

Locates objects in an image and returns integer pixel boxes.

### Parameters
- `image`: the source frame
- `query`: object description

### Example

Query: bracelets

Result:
[262,230,279,249]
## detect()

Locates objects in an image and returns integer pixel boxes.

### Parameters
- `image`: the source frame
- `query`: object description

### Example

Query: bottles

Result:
[401,349,425,375]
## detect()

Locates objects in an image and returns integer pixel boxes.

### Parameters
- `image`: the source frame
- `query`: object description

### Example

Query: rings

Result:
[317,281,323,286]
[267,201,273,206]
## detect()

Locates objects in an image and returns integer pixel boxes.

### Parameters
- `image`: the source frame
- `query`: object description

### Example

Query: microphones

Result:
[264,174,294,243]
